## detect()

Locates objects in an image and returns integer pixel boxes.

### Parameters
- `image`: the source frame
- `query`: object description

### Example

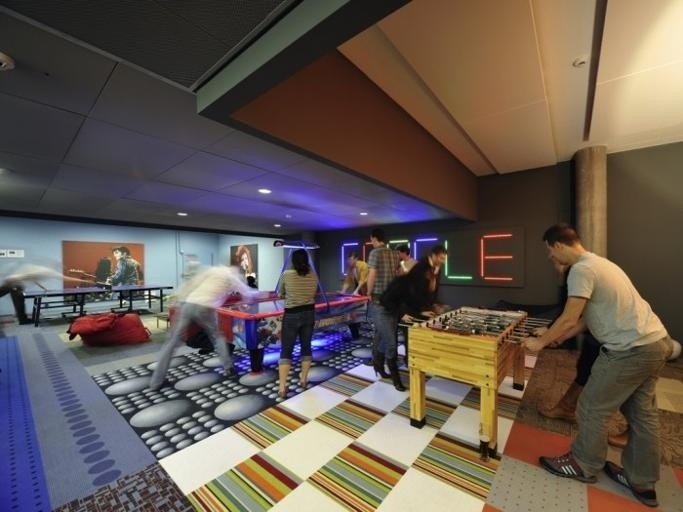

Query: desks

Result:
[167,291,279,342]
[216,292,370,375]
[21,285,106,327]
[110,283,174,314]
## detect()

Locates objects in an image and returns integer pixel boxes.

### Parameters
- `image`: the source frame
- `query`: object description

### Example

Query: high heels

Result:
[388,359,405,391]
[371,349,389,378]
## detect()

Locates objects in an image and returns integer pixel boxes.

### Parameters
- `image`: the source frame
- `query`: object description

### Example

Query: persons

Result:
[370,260,432,390]
[239,249,250,272]
[528,251,638,448]
[147,261,258,390]
[335,253,371,301]
[105,247,141,286]
[394,244,419,272]
[408,245,453,315]
[535,220,674,506]
[365,227,405,362]
[273,248,320,398]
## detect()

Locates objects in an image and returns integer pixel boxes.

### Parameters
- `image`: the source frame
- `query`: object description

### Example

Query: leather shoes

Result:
[609,428,630,447]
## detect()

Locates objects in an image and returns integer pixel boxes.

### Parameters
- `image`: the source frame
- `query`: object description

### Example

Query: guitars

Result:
[69,268,143,287]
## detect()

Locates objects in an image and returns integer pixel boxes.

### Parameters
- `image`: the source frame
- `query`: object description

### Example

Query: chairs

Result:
[152,294,174,328]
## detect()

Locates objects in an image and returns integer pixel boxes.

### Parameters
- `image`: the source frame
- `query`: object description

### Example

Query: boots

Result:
[538,382,584,423]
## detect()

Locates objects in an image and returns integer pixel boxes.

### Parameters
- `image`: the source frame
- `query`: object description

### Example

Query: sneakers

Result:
[539,452,597,483]
[604,461,657,507]
